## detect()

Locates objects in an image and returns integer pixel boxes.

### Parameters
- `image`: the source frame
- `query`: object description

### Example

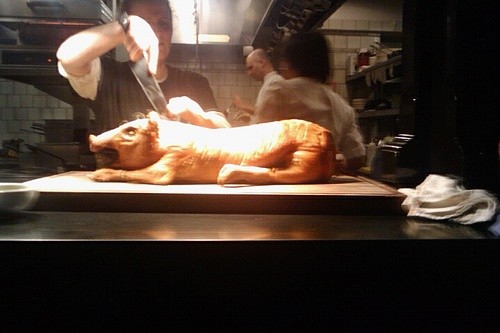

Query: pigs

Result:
[87,109,337,186]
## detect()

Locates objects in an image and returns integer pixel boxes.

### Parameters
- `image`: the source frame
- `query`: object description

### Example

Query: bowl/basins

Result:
[352,98,366,110]
[0,183,34,212]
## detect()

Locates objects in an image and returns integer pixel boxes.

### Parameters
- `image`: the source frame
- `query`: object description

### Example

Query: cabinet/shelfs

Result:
[345,48,402,122]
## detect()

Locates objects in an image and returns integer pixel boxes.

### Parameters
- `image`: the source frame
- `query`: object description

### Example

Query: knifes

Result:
[118,12,168,116]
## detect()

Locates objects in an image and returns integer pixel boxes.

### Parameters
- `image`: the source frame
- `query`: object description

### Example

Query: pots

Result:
[19,119,80,169]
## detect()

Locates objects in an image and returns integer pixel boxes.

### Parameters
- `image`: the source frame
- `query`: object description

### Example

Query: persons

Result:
[230,47,286,124]
[259,29,368,176]
[55,0,232,169]
